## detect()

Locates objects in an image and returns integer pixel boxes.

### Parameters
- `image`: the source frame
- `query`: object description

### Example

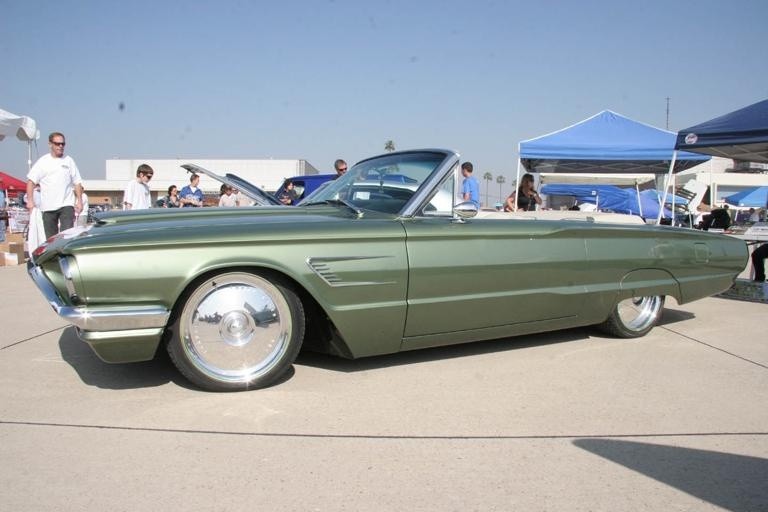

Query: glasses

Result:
[52,142,65,146]
[147,175,151,180]
[339,167,347,171]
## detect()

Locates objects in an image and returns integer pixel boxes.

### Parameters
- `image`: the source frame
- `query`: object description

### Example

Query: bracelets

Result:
[531,192,537,197]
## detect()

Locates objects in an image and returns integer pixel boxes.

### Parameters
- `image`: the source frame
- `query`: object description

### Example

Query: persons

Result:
[123,164,153,209]
[23,183,47,257]
[327,159,356,201]
[750,244,768,286]
[155,173,205,207]
[74,186,89,226]
[0,177,9,243]
[736,207,765,225]
[278,179,298,204]
[711,205,731,230]
[461,162,480,211]
[506,173,542,212]
[218,184,257,207]
[504,200,514,212]
[26,132,84,240]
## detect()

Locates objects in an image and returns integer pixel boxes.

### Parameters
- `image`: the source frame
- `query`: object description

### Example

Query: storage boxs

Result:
[0,233,24,266]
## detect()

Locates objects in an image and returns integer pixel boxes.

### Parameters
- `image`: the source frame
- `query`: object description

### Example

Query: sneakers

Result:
[751,281,766,286]
